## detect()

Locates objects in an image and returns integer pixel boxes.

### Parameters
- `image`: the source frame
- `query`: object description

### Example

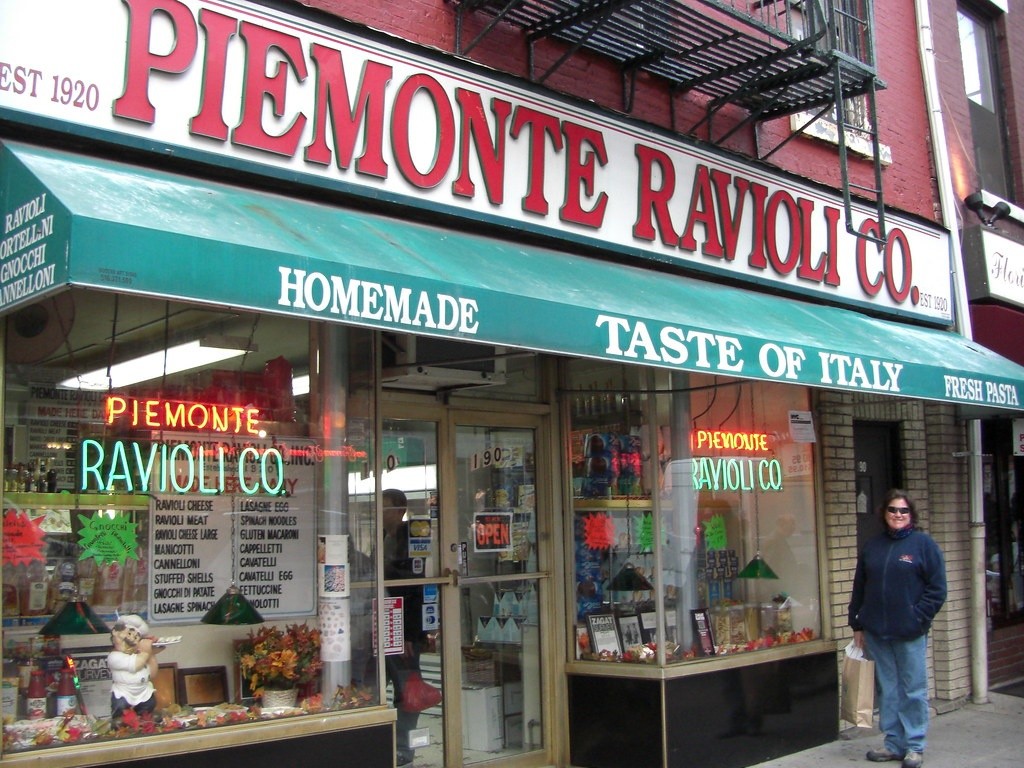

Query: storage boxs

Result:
[0,662,21,724]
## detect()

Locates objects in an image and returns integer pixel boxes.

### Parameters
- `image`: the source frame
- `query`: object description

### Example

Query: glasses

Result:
[887,506,911,514]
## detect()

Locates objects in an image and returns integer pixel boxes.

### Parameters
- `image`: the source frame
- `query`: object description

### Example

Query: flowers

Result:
[233,620,321,699]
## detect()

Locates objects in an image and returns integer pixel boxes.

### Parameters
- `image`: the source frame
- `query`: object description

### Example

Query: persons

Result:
[625,623,638,644]
[343,489,427,768]
[106,614,159,727]
[845,490,949,768]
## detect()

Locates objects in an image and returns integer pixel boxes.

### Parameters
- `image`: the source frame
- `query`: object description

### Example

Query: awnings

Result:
[0,140,1024,422]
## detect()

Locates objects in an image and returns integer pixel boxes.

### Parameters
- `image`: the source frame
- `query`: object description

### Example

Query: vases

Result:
[261,688,301,717]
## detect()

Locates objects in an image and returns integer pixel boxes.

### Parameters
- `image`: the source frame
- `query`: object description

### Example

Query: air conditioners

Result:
[380,329,508,395]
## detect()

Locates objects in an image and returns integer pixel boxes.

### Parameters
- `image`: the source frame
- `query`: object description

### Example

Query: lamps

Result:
[54,334,258,393]
[605,363,653,591]
[964,191,1010,226]
[198,432,262,624]
[736,380,779,579]
[38,374,112,634]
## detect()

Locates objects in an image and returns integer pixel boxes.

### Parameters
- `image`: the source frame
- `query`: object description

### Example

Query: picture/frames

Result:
[59,644,229,720]
[574,607,716,662]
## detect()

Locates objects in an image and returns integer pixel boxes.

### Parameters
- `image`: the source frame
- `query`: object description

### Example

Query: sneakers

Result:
[902,752,923,768]
[867,747,905,762]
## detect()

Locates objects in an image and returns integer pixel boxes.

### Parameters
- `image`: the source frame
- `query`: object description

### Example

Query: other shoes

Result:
[396,750,413,768]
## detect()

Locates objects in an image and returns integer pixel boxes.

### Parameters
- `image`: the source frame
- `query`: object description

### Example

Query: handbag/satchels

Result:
[402,671,442,713]
[840,646,875,729]
